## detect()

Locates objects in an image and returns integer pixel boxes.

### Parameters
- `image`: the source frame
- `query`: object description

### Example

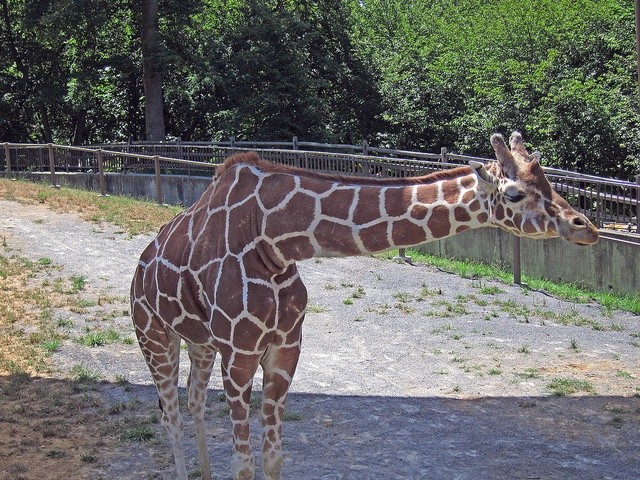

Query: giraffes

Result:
[128,131,601,480]
